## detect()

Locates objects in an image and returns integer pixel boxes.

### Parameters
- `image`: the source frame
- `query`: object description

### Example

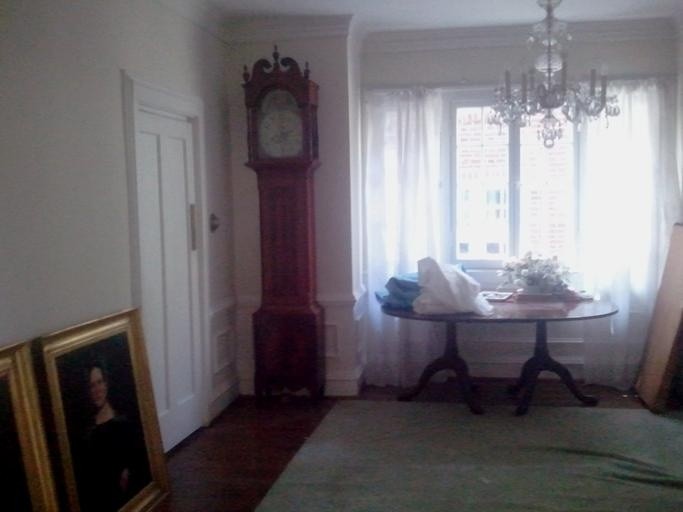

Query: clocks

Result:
[240,43,321,176]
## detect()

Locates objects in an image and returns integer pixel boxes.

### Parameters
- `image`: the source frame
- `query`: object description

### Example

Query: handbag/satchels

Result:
[412,257,479,316]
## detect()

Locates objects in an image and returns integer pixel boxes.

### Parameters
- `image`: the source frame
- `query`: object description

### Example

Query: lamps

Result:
[485,1,621,150]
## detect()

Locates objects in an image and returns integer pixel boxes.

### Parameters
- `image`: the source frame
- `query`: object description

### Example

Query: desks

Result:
[378,298,620,417]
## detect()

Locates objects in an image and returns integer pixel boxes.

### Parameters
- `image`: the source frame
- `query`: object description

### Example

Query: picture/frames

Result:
[0,304,173,512]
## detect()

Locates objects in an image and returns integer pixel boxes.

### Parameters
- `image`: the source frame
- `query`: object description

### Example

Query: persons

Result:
[71,360,149,512]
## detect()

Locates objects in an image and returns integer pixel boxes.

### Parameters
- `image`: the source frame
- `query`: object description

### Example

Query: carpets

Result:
[252,402,683,512]
